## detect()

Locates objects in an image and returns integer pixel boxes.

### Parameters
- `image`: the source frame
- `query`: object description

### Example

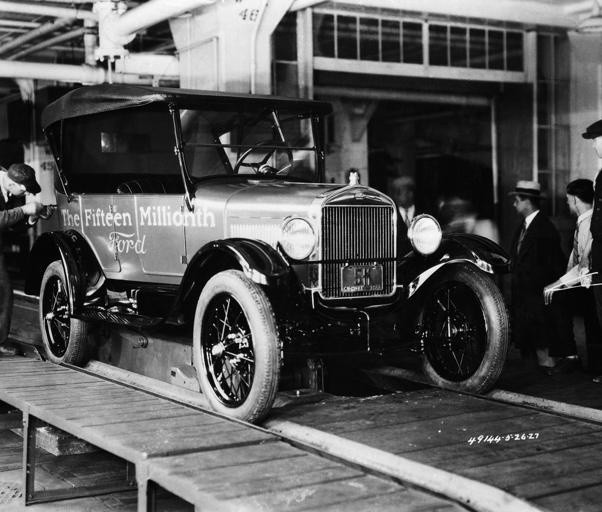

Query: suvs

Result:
[25,84,513,424]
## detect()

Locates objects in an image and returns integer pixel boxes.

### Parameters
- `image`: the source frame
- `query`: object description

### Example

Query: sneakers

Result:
[513,356,601,384]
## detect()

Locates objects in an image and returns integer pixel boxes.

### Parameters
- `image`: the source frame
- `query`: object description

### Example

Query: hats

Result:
[582,119,602,139]
[565,179,594,196]
[508,181,548,199]
[7,163,41,193]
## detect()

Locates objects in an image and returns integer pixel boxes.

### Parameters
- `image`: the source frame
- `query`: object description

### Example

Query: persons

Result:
[488,179,565,377]
[435,194,502,248]
[1,162,56,357]
[385,166,425,264]
[522,178,602,382]
[579,119,602,386]
